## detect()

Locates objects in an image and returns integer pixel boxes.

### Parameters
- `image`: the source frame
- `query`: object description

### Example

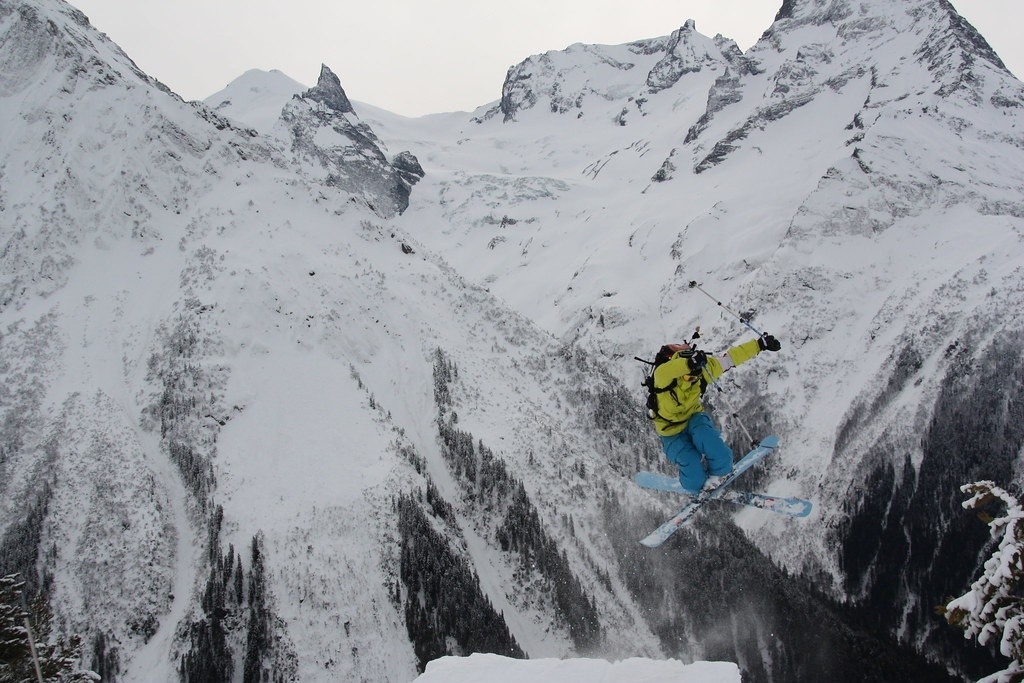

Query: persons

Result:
[647,332,782,491]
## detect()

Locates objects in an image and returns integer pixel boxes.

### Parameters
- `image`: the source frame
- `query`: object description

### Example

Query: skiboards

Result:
[635,434,813,550]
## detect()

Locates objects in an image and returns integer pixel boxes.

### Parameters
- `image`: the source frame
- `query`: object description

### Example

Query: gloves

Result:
[759,336,782,352]
[689,350,708,369]
